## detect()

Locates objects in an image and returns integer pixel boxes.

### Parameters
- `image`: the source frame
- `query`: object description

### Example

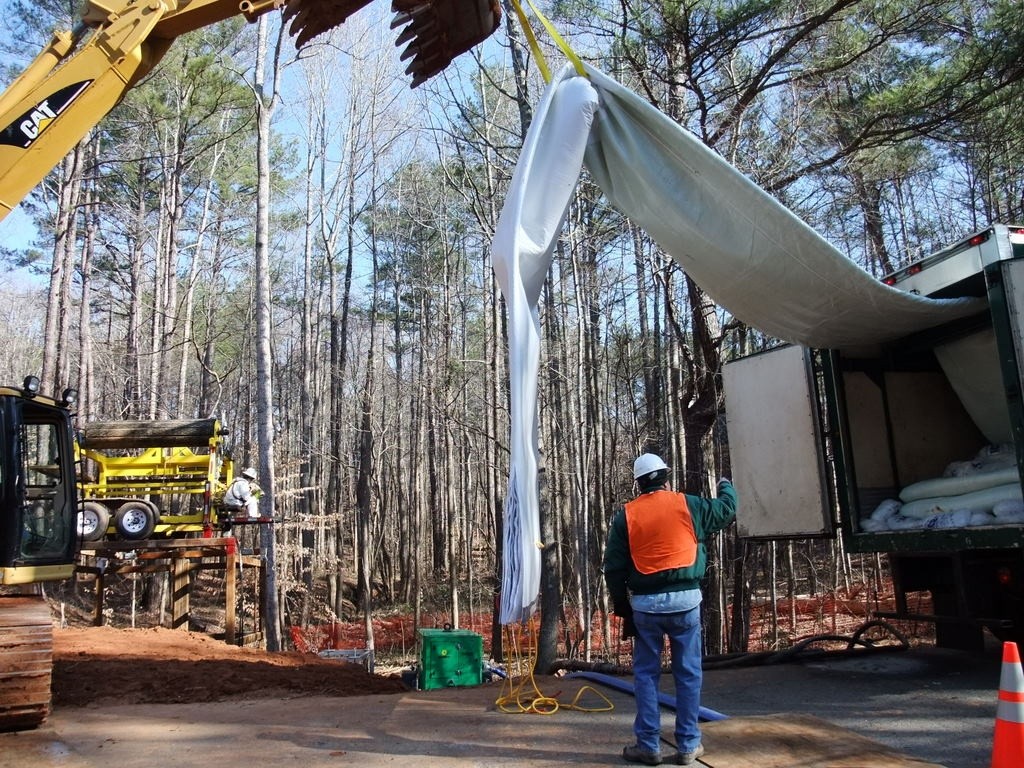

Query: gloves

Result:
[611,599,639,643]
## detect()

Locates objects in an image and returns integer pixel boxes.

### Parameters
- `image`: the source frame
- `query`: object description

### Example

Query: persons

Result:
[603,452,737,765]
[224,468,265,517]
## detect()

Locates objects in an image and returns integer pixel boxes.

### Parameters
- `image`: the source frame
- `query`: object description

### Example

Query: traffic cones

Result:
[986,641,1024,768]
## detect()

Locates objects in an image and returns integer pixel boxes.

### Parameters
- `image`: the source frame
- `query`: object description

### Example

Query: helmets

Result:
[632,452,673,483]
[241,468,258,479]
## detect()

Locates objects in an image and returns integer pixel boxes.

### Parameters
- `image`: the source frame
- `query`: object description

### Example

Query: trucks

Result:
[711,221,1023,656]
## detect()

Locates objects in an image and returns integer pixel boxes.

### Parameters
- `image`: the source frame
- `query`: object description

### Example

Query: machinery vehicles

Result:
[0,2,507,602]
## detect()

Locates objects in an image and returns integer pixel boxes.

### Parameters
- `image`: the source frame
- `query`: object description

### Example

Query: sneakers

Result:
[675,742,705,765]
[624,743,662,765]
